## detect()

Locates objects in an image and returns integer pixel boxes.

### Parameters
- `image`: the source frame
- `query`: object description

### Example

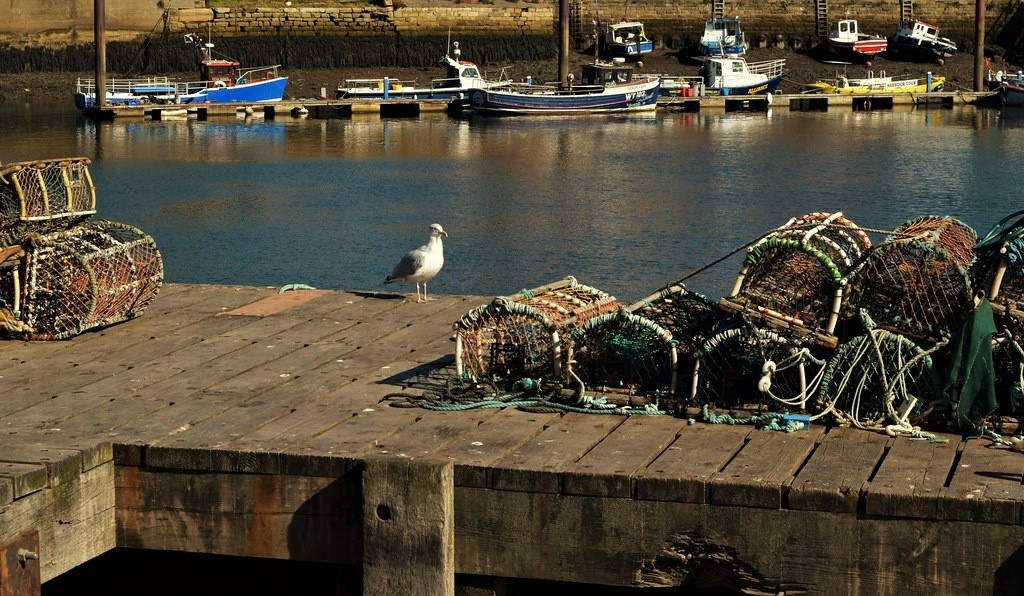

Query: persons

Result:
[567,68,574,91]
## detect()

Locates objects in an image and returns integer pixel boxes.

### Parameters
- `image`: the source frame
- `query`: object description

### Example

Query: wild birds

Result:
[382,223,449,305]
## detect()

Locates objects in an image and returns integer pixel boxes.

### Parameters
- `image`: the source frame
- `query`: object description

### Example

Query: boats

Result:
[468,77,660,114]
[804,70,945,95]
[605,17,786,96]
[986,69,1024,104]
[826,18,958,58]
[74,33,513,110]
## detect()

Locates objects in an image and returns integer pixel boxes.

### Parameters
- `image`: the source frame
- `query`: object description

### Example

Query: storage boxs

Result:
[721,88,730,96]
[393,84,403,91]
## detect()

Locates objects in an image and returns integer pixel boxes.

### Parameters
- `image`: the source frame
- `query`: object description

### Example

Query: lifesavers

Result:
[621,17,630,23]
[213,81,228,88]
[459,60,474,65]
